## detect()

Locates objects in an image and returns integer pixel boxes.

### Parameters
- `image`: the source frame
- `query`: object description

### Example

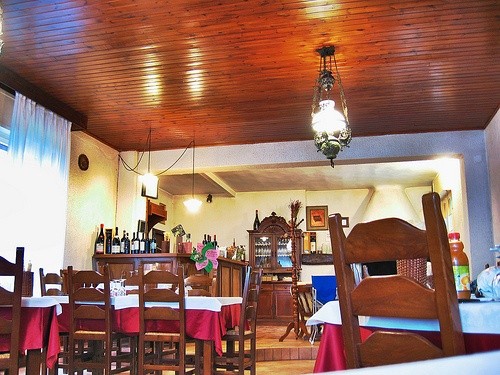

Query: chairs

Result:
[0,192,465,375]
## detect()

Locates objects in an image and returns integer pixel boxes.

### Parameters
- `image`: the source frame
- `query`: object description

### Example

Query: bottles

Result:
[492,244,500,301]
[95,223,158,254]
[202,233,218,250]
[254,210,260,230]
[232,237,246,262]
[310,233,316,254]
[448,232,471,300]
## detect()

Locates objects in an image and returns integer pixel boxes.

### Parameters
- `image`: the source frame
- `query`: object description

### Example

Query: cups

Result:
[255,238,292,255]
[110,278,126,299]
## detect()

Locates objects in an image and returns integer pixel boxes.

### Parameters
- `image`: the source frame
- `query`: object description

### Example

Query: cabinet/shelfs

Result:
[245,213,303,325]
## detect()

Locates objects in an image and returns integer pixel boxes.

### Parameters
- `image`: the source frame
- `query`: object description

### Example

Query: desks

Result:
[306,302,500,373]
[0,294,63,375]
[44,296,255,375]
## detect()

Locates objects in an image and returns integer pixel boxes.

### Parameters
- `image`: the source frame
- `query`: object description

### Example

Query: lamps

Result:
[311,45,352,168]
[118,130,212,212]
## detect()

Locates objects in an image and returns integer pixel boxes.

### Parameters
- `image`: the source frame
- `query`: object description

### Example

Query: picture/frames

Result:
[306,206,328,230]
